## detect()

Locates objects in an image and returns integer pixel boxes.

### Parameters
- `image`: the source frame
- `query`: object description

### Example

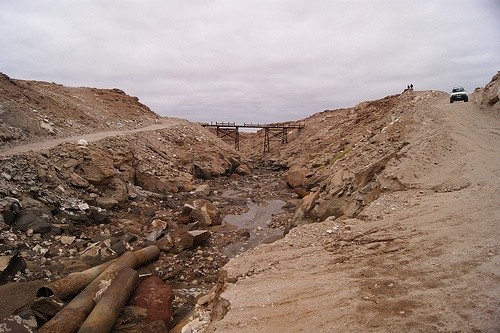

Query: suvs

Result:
[450,87,469,103]
